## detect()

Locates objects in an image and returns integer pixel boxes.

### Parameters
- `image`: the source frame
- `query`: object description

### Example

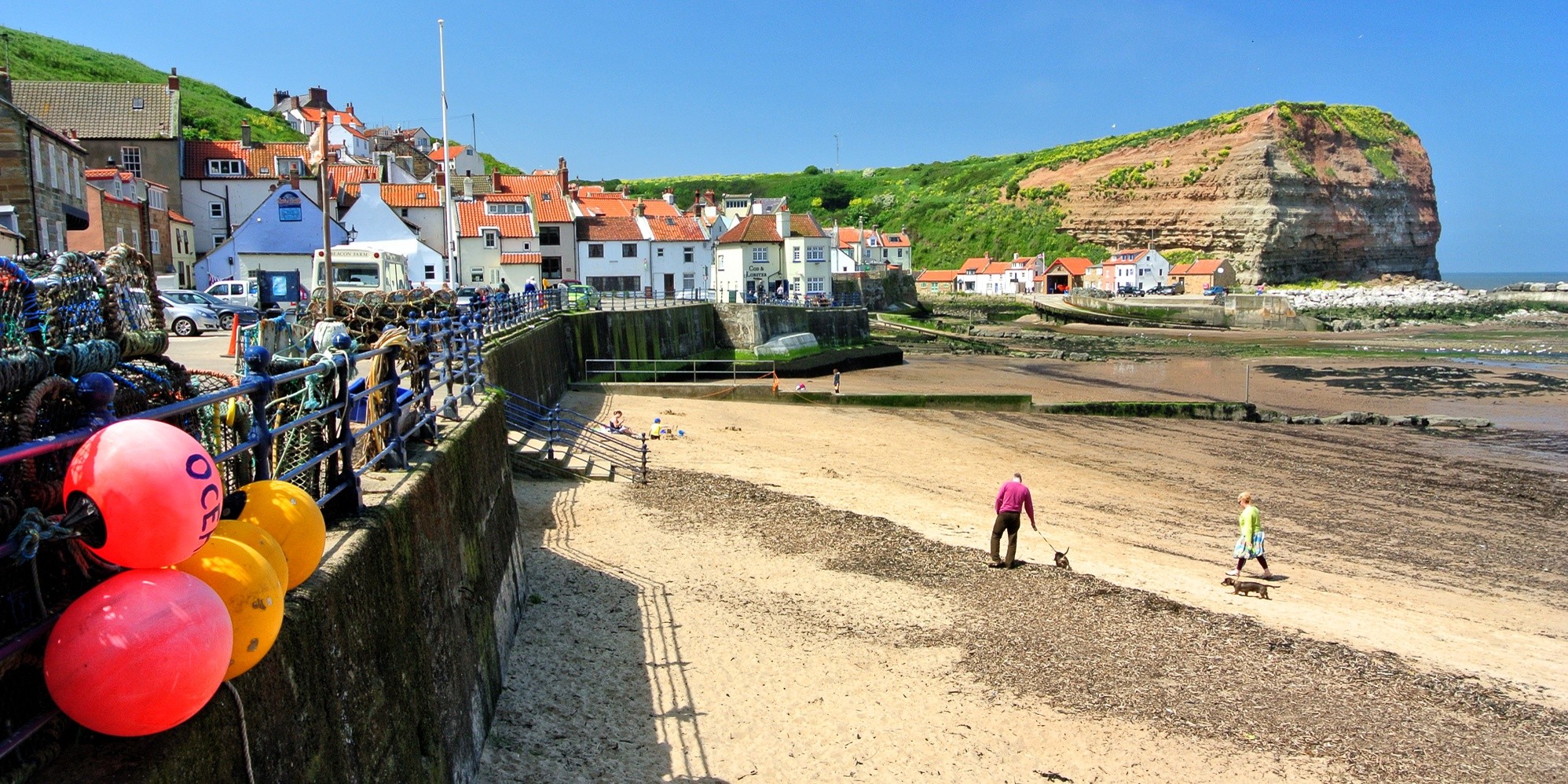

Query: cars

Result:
[1116,286,1145,299]
[125,288,219,337]
[1148,283,1179,295]
[1204,285,1228,297]
[566,284,600,312]
[455,284,496,315]
[159,289,259,334]
[560,278,581,289]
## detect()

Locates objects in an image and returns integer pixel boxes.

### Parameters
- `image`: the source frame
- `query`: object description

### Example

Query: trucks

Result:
[311,248,408,312]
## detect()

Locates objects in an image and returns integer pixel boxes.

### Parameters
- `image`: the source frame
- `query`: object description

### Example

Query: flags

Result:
[308,126,322,165]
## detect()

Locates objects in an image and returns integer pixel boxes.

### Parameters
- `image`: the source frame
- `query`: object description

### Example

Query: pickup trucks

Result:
[202,281,260,310]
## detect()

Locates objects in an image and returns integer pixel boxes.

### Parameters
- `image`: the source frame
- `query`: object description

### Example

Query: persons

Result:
[833,368,840,393]
[648,417,670,440]
[609,410,638,435]
[1225,491,1273,579]
[749,279,842,309]
[473,275,541,326]
[796,382,806,390]
[987,472,1037,569]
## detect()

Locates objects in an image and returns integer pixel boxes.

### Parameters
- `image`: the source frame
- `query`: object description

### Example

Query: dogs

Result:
[1054,547,1072,570]
[1220,578,1281,599]
